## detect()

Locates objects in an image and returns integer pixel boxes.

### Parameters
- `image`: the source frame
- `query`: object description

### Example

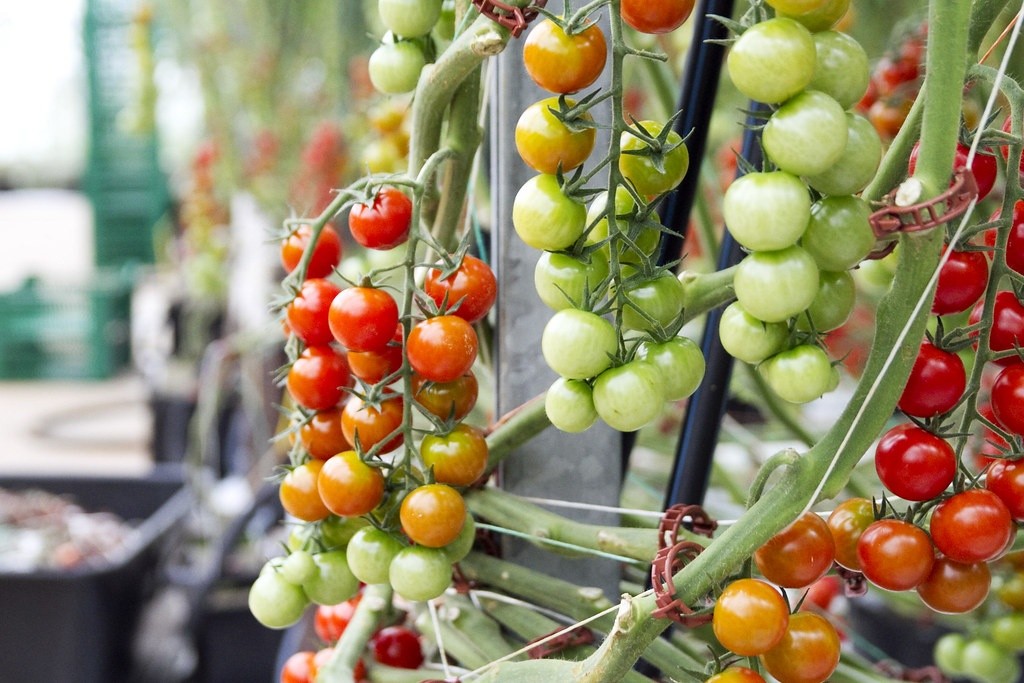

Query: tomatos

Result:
[247,0,1024,683]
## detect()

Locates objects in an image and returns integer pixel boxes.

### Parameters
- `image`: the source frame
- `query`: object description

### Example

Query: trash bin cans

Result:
[0,470,203,683]
